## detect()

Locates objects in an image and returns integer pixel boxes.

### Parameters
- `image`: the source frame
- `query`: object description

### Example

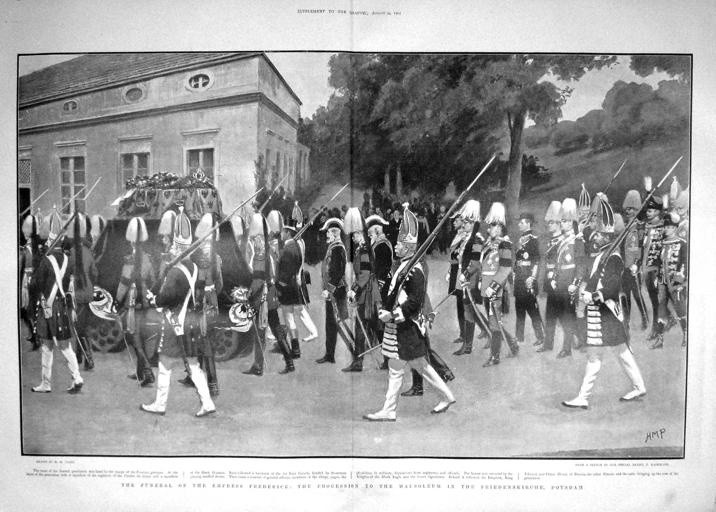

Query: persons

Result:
[20,184,688,422]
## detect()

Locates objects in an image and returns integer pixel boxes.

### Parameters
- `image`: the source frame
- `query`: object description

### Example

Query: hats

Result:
[21,209,299,245]
[318,201,419,260]
[451,176,690,227]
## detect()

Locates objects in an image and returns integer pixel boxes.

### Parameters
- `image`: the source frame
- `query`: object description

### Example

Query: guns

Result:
[152,189,262,297]
[568,155,684,318]
[385,154,496,311]
[268,184,348,286]
[21,178,100,283]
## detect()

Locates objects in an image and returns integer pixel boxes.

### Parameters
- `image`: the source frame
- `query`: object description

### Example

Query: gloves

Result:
[378,309,391,323]
[320,290,357,301]
[629,263,637,274]
[445,261,592,306]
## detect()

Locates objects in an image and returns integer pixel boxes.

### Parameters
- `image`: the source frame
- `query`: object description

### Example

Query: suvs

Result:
[69,168,260,365]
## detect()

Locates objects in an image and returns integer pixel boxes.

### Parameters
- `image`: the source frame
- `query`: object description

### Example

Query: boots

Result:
[25,317,94,393]
[316,318,455,422]
[126,332,218,417]
[453,295,687,368]
[242,305,318,375]
[618,348,647,401]
[562,358,601,410]
